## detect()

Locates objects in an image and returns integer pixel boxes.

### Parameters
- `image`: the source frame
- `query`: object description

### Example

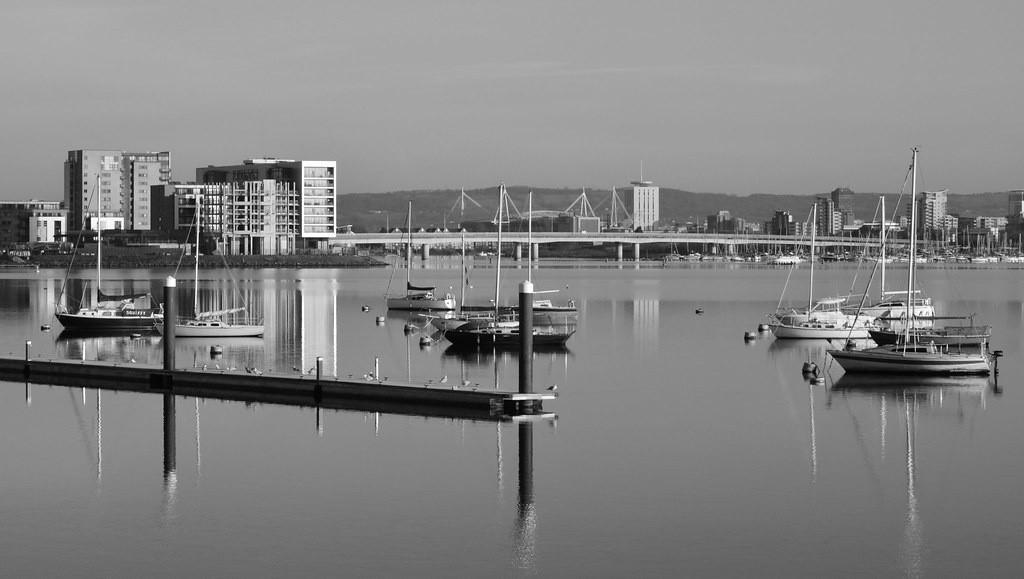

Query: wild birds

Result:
[130,356,136,363]
[202,358,471,391]
[546,382,558,394]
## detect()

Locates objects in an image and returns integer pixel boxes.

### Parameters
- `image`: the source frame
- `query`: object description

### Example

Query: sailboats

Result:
[828,374,990,526]
[55,329,162,480]
[841,196,935,331]
[661,235,685,260]
[160,337,264,469]
[825,147,990,376]
[867,201,991,349]
[765,202,880,338]
[680,229,761,262]
[53,174,164,333]
[418,185,579,343]
[386,309,456,383]
[384,200,456,310]
[152,202,265,337]
[948,225,1024,263]
